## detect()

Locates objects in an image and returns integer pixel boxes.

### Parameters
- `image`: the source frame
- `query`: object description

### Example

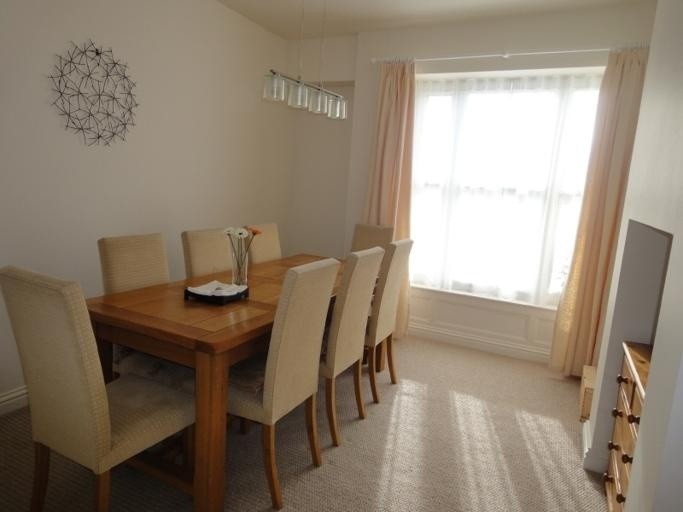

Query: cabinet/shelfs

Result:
[602,339,653,511]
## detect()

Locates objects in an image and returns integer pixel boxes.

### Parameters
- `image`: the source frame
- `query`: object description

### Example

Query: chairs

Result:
[98,231,193,390]
[179,223,412,509]
[0,265,196,510]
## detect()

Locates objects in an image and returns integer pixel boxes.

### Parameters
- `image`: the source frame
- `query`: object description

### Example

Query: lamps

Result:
[259,1,347,122]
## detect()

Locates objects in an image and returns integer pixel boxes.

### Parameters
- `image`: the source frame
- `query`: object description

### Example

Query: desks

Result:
[85,253,348,509]
